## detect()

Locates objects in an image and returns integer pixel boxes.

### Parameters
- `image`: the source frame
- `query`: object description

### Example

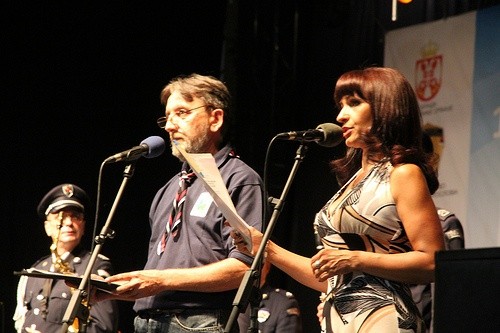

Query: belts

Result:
[140,305,226,317]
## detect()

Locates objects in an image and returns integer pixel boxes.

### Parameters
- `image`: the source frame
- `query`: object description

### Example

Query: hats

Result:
[36,183,89,216]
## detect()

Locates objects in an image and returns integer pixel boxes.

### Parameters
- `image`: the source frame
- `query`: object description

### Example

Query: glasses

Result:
[157,103,214,129]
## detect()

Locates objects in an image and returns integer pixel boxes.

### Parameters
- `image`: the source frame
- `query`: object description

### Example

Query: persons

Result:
[224,67,445,333]
[237,259,303,333]
[65,74,271,333]
[13,184,118,333]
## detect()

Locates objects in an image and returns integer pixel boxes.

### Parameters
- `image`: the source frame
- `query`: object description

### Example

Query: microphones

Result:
[276,123,343,146]
[107,136,165,163]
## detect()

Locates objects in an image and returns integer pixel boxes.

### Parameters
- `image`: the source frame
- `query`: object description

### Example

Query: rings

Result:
[314,260,321,268]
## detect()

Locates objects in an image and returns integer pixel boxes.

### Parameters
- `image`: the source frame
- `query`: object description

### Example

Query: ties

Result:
[156,147,236,256]
